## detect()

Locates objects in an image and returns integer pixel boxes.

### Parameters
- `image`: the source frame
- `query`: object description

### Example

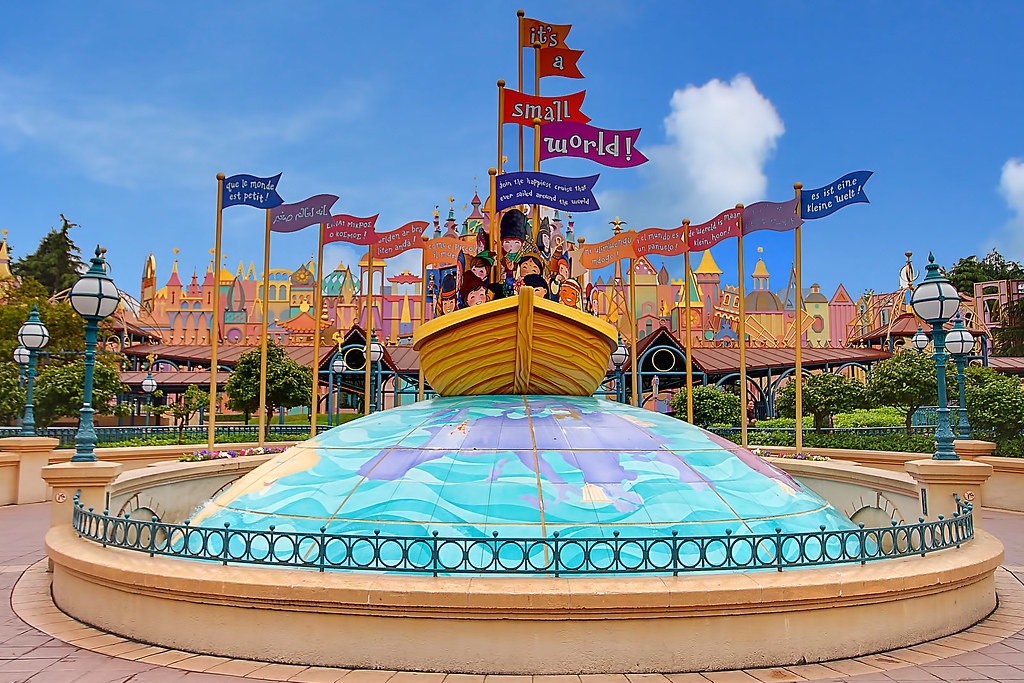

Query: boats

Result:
[413,286,618,397]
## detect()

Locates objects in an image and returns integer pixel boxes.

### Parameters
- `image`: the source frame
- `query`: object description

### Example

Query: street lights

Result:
[16,304,51,437]
[69,244,121,462]
[332,352,347,426]
[13,346,30,427]
[610,331,630,402]
[909,251,961,460]
[363,328,384,413]
[911,322,929,353]
[944,312,976,440]
[141,372,157,426]
